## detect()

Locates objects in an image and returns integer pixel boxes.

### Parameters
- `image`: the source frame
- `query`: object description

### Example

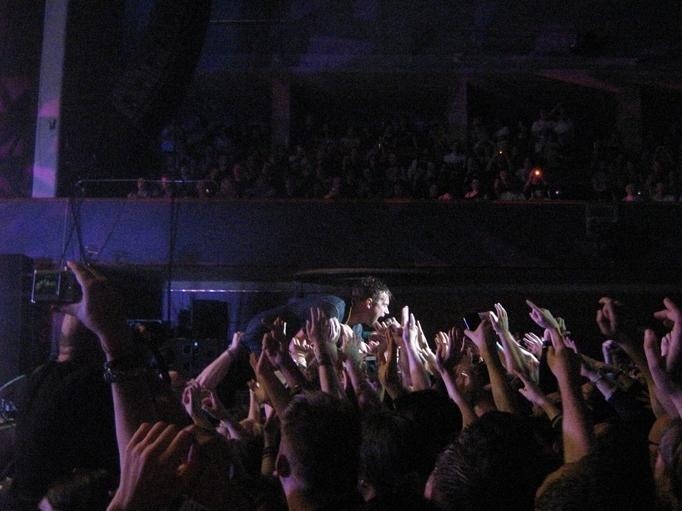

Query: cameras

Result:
[31,269,82,304]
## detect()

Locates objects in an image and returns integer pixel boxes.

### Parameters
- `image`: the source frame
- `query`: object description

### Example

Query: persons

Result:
[1,254,156,511]
[532,326,626,511]
[348,408,433,511]
[271,391,353,511]
[157,275,682,416]
[421,410,559,510]
[103,416,199,511]
[653,416,682,510]
[125,102,682,207]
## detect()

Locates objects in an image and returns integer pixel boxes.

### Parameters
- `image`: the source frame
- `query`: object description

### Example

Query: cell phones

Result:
[462,313,482,331]
